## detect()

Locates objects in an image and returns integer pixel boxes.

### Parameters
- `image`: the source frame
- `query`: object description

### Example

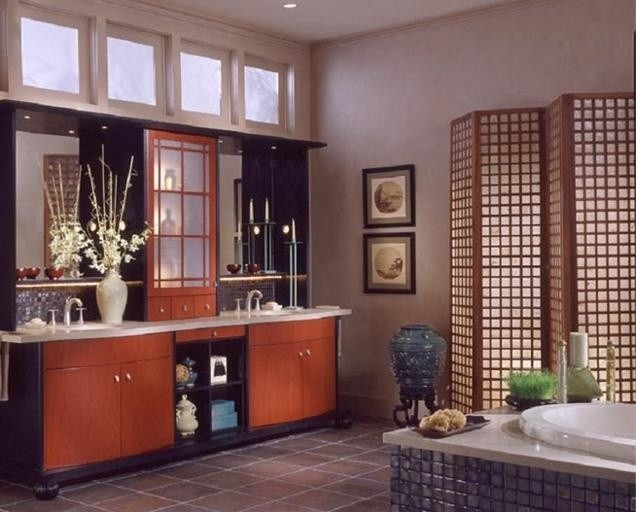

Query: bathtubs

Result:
[518,401,636,461]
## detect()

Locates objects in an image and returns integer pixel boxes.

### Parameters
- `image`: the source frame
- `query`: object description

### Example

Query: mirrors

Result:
[0,98,148,280]
[217,129,328,276]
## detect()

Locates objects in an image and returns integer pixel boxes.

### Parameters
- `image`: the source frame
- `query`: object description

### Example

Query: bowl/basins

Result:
[226,263,241,273]
[45,266,64,280]
[16,267,40,281]
[246,264,259,275]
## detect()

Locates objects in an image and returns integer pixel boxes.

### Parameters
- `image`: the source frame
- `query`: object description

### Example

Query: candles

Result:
[570,332,589,366]
[238,222,242,240]
[291,218,296,242]
[265,197,269,220]
[249,198,254,220]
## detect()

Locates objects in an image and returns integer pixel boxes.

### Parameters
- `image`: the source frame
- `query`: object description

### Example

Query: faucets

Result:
[62,297,83,327]
[244,289,263,312]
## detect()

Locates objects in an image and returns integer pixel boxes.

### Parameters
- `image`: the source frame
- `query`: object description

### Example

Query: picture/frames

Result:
[362,232,417,295]
[234,178,242,232]
[233,237,242,265]
[362,164,415,228]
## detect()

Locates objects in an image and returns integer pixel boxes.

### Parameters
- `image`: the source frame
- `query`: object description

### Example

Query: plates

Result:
[26,321,48,329]
[412,414,490,439]
[261,304,282,311]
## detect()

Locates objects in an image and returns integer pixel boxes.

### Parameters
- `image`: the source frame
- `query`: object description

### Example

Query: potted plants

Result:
[504,369,556,410]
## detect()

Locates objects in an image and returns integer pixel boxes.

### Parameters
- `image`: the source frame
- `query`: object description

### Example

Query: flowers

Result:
[39,163,92,281]
[74,143,150,274]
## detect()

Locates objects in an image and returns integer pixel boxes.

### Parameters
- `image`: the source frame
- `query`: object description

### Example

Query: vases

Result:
[162,209,176,233]
[385,324,447,389]
[164,168,176,190]
[95,270,128,324]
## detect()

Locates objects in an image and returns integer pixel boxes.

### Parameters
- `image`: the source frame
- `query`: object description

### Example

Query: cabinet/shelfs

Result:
[248,317,336,439]
[7,332,175,487]
[175,324,248,458]
[146,129,218,319]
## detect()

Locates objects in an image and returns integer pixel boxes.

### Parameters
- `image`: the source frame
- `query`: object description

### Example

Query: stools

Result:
[392,389,440,428]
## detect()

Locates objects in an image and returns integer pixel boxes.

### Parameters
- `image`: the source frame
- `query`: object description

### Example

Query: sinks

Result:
[52,320,121,330]
[249,310,294,316]
